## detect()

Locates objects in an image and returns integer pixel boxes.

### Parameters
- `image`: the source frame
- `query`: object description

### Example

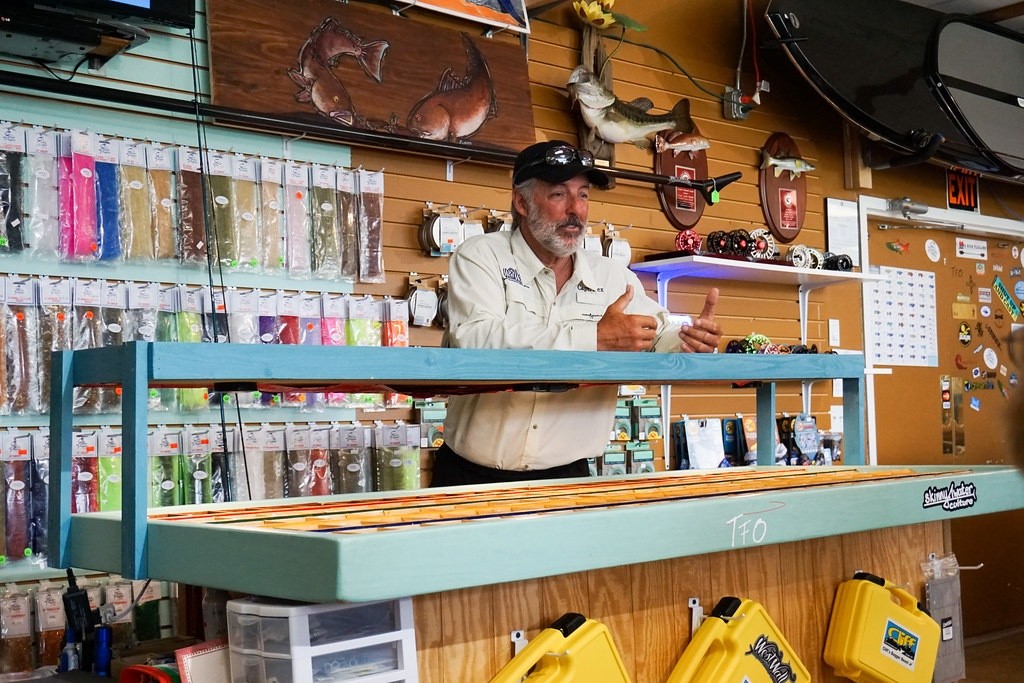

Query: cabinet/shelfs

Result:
[224,596,419,683]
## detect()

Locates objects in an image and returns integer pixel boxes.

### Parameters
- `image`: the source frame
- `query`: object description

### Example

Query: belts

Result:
[439,443,587,480]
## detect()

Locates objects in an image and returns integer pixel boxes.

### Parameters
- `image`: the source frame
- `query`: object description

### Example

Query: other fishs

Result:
[760,147,816,181]
[566,64,694,151]
[655,128,710,159]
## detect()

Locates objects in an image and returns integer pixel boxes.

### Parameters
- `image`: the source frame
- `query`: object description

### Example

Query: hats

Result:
[512,139,610,186]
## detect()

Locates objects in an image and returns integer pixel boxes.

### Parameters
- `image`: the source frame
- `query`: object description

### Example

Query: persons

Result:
[431,140,723,491]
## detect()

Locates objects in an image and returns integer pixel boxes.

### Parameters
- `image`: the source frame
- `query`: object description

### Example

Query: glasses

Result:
[523,146,596,169]
